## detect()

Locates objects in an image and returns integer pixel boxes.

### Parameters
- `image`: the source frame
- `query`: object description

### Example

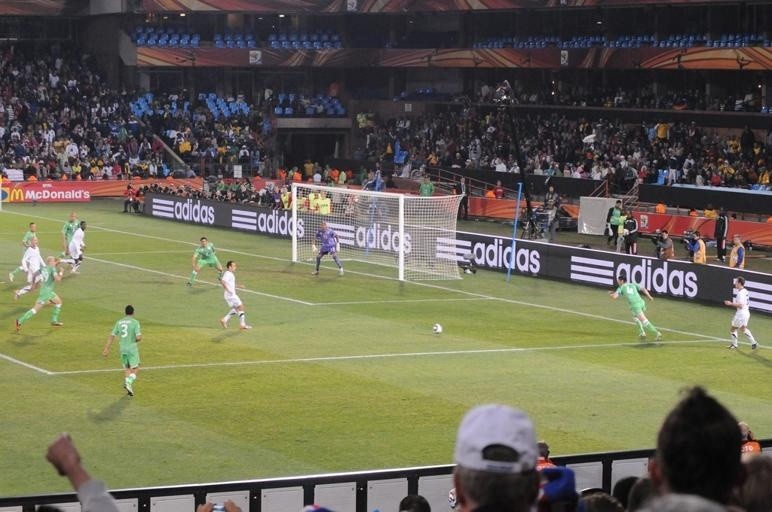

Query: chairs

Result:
[274,94,347,115]
[394,88,436,101]
[213,26,257,48]
[751,182,772,191]
[161,164,172,176]
[132,93,250,123]
[474,35,770,49]
[267,28,343,49]
[656,169,669,184]
[394,150,408,165]
[129,25,200,48]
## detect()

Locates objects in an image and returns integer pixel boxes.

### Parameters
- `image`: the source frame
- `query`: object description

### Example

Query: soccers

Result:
[433,324,442,333]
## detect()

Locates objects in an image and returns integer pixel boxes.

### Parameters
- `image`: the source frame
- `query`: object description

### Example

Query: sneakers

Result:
[51,322,63,326]
[752,342,759,349]
[657,334,662,341]
[312,270,320,275]
[639,333,646,336]
[337,270,344,277]
[16,319,21,330]
[240,325,251,329]
[123,383,134,396]
[220,318,227,328]
[725,343,737,348]
[15,290,18,301]
[9,273,14,283]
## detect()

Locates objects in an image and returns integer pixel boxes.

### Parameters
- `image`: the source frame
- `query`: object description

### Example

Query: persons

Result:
[9,222,38,283]
[310,222,344,277]
[14,255,63,331]
[195,499,242,512]
[0,39,771,272]
[187,237,225,289]
[102,305,143,397]
[723,276,760,350]
[58,220,87,276]
[13,237,47,301]
[44,434,122,512]
[385,382,772,511]
[607,275,663,342]
[218,260,252,330]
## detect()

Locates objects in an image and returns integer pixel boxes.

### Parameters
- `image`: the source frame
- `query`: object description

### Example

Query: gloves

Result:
[311,244,318,253]
[336,243,340,253]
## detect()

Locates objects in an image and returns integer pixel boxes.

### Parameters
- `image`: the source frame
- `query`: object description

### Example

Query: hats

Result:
[454,404,538,473]
